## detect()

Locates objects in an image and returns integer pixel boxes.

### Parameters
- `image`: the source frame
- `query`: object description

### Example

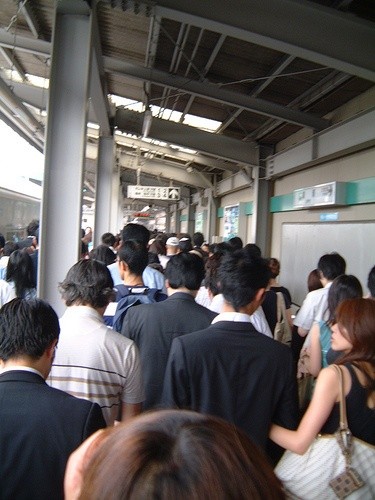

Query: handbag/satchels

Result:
[274,292,292,348]
[270,364,375,500]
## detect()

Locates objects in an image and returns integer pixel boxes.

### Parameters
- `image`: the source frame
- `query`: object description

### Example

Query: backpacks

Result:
[112,284,162,334]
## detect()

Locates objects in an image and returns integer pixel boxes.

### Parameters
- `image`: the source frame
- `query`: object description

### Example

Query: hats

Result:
[166,237,179,245]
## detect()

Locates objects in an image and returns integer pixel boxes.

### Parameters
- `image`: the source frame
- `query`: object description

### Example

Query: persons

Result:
[45,259,147,428]
[0,220,375,500]
